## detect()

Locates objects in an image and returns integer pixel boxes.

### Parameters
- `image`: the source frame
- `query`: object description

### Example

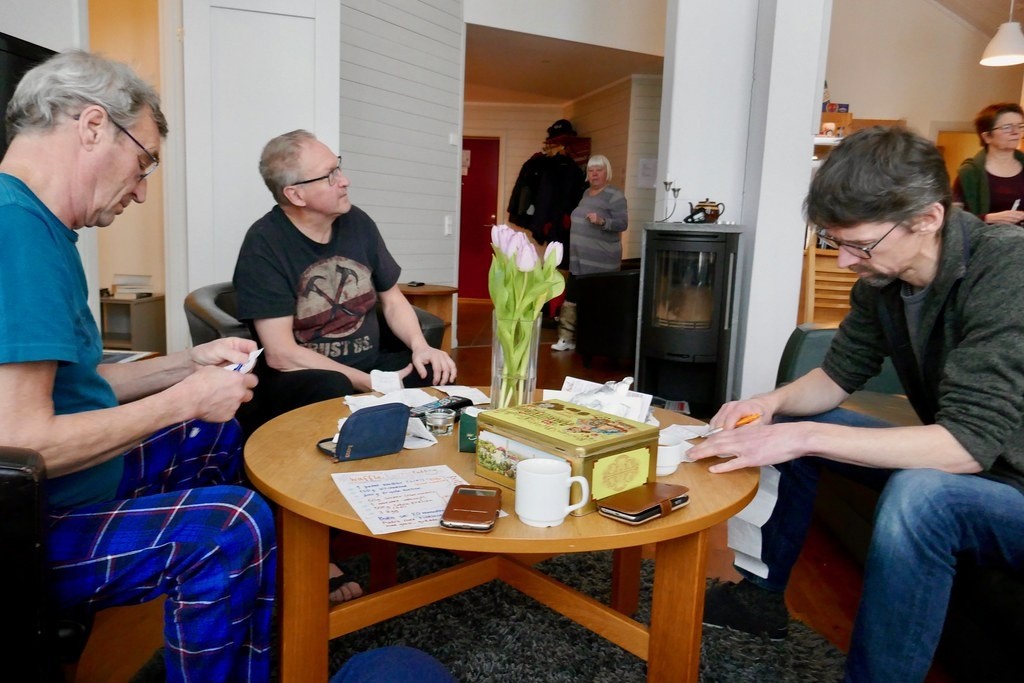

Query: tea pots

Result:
[688,198,725,223]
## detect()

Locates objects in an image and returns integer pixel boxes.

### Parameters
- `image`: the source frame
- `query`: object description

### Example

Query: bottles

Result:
[837,126,845,138]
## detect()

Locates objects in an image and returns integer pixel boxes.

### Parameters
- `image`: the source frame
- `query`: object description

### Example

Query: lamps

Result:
[979,0,1024,68]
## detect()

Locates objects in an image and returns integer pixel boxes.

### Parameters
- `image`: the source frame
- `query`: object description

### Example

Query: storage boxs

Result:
[472,398,660,519]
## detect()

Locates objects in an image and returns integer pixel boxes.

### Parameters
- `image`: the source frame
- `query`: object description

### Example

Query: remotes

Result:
[408,395,486,427]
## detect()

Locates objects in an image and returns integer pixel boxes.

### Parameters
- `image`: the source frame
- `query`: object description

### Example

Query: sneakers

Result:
[702,575,788,642]
[550,338,576,351]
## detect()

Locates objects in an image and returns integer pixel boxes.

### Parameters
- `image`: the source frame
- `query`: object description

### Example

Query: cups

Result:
[514,459,590,528]
[822,123,836,135]
[655,436,683,476]
[425,408,456,434]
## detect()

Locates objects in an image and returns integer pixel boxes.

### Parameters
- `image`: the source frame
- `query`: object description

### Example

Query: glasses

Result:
[72,108,161,183]
[983,123,1024,133]
[290,156,343,186]
[814,218,905,260]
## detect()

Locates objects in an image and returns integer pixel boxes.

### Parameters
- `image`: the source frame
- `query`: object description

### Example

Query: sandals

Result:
[328,558,365,604]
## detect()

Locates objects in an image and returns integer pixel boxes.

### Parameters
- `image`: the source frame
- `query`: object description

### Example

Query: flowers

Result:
[488,224,565,407]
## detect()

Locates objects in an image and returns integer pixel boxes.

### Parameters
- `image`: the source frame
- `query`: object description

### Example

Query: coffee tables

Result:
[245,387,762,683]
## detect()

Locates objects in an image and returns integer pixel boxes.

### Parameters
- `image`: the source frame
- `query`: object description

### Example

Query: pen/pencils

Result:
[1011,199,1020,210]
[700,411,766,437]
[188,362,243,440]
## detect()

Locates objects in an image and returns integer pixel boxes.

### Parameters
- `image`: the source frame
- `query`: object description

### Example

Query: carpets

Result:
[126,549,849,683]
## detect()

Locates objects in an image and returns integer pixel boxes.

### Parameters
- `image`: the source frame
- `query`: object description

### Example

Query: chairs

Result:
[0,277,1024,683]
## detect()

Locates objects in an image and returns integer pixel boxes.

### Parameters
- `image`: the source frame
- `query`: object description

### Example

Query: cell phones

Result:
[600,494,688,521]
[440,485,503,531]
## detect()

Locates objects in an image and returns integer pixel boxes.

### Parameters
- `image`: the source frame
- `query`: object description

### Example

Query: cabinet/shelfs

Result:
[399,283,458,356]
[796,114,908,324]
[96,293,166,353]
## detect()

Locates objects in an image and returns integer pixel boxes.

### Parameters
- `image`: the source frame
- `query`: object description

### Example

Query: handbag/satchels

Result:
[318,403,410,463]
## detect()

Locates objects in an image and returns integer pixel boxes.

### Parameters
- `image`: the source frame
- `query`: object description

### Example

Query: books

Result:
[110,274,154,300]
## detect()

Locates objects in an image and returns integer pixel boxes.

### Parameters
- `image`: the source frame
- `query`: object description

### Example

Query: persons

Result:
[229,130,456,407]
[570,154,626,358]
[685,129,1024,683]
[0,50,279,683]
[952,103,1024,231]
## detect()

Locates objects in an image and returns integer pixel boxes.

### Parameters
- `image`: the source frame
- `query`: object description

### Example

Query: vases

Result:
[488,308,543,410]
